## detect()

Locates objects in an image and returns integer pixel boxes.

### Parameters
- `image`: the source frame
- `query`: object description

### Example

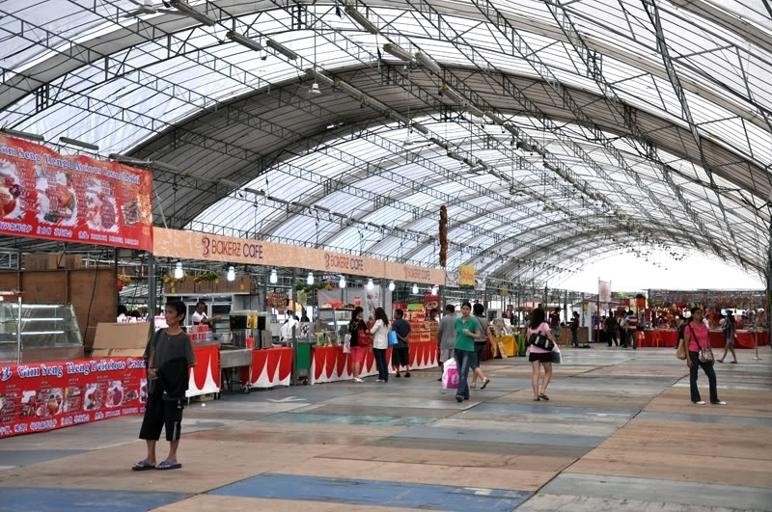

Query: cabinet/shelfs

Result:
[1,302,86,361]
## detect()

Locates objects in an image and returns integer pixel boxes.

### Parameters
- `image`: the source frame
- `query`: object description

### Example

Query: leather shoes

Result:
[456,393,464,403]
[464,395,469,400]
[405,373,410,377]
[396,373,400,377]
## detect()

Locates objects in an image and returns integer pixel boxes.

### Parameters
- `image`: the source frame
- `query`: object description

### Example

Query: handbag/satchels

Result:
[677,339,687,360]
[528,330,554,351]
[357,329,374,347]
[699,348,715,363]
[388,330,399,346]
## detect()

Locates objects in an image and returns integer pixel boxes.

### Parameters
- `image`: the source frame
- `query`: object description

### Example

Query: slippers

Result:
[538,392,549,400]
[534,397,541,401]
[132,459,157,471]
[155,459,182,470]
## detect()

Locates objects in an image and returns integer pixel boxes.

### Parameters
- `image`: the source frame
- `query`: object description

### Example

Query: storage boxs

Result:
[91,321,153,359]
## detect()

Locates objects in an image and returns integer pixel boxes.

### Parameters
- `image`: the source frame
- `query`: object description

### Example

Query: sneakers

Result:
[352,376,365,383]
[713,399,727,405]
[694,399,705,404]
[480,376,490,389]
[376,378,385,383]
[470,382,477,388]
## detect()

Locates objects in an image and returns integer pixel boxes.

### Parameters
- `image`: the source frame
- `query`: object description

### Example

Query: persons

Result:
[601,306,645,349]
[741,309,768,329]
[569,311,579,348]
[649,302,712,330]
[391,308,412,378]
[452,301,481,401]
[682,305,726,407]
[191,301,223,341]
[432,305,459,382]
[469,303,498,391]
[717,310,737,363]
[301,309,311,323]
[524,308,560,402]
[348,305,370,383]
[369,307,392,383]
[538,302,558,339]
[130,297,196,473]
[283,309,299,326]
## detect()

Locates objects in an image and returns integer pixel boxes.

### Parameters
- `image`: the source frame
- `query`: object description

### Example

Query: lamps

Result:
[1,129,151,168]
[173,260,439,295]
[153,0,687,272]
